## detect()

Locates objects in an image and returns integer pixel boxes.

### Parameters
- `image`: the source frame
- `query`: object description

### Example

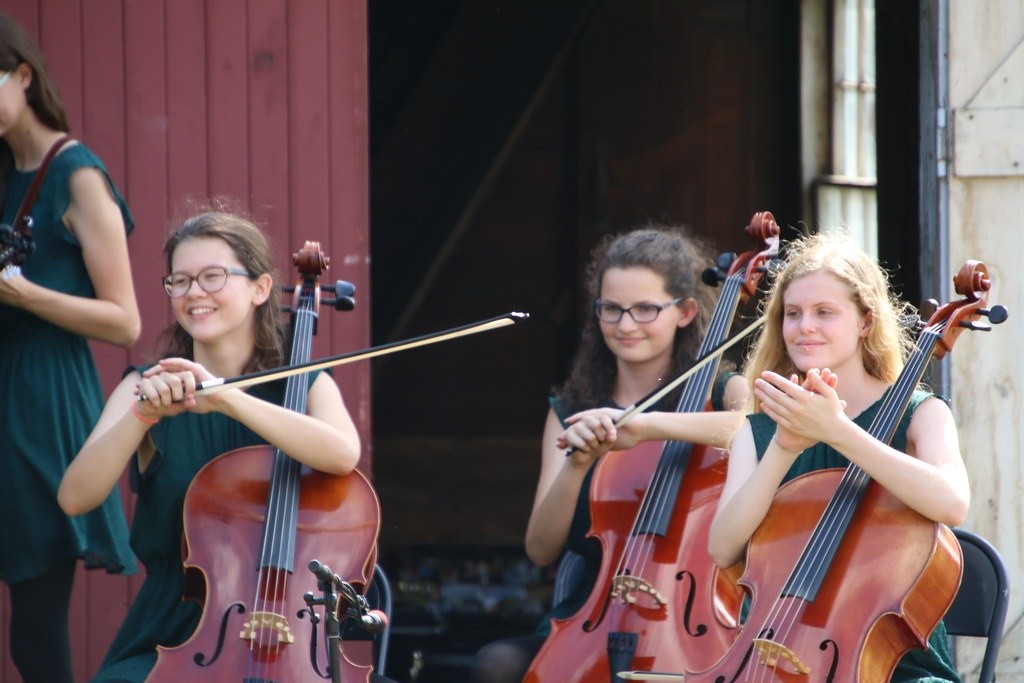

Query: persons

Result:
[0,19,144,683]
[708,234,969,682]
[56,213,361,681]
[524,226,757,683]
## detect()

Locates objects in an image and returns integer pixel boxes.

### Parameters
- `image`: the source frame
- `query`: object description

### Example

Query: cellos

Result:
[522,209,782,682]
[139,234,383,683]
[678,258,1006,683]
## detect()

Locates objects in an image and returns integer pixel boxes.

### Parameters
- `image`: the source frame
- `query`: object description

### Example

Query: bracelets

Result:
[131,398,158,428]
[772,435,803,456]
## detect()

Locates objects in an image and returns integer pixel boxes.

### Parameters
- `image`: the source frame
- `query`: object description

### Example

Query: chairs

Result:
[943,529,1011,683]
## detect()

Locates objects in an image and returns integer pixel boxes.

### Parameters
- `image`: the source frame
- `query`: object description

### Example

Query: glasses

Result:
[594,297,682,323]
[161,266,250,298]
